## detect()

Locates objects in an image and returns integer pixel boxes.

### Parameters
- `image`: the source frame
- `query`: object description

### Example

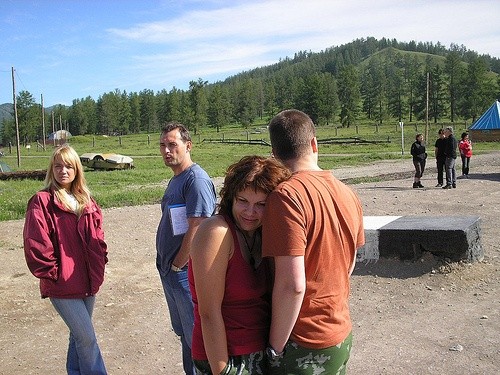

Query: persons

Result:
[411,134,428,189]
[434,128,448,187]
[188,155,293,375]
[262,109,366,375]
[458,132,472,178]
[156,123,216,375]
[23,142,109,375]
[442,126,457,189]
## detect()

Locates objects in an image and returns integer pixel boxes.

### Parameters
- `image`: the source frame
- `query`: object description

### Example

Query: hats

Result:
[447,126,453,133]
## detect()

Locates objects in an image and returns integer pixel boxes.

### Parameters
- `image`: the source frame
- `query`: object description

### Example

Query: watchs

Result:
[264,343,285,361]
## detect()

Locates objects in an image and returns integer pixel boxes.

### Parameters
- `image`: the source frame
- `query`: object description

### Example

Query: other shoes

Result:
[452,184,456,188]
[418,181,424,187]
[413,182,419,188]
[442,184,452,189]
[466,175,470,178]
[435,183,442,187]
[463,174,466,178]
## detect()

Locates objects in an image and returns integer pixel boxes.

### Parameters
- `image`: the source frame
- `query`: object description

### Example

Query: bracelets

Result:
[171,264,183,272]
